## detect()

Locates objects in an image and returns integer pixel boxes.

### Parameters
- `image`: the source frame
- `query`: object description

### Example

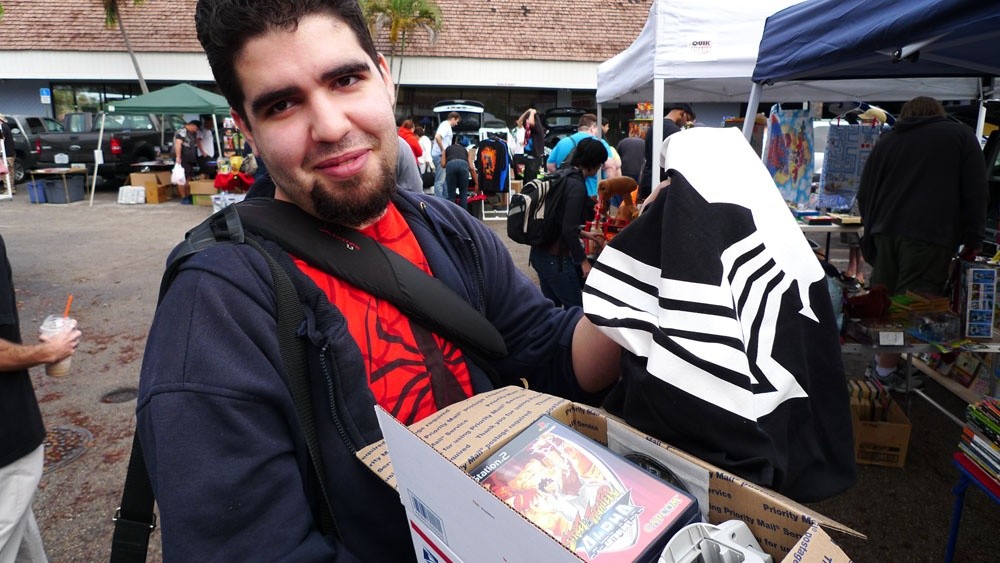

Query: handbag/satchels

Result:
[421,165,436,189]
[243,153,258,175]
[170,162,186,185]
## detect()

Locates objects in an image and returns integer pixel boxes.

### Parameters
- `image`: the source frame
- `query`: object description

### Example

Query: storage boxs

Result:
[188,179,221,206]
[210,192,246,212]
[846,384,912,469]
[354,385,868,563]
[500,179,522,206]
[130,171,174,204]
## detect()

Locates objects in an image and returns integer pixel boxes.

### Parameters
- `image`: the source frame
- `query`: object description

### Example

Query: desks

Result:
[941,452,1000,563]
[129,159,175,198]
[790,211,863,263]
[30,167,89,206]
[843,311,1000,428]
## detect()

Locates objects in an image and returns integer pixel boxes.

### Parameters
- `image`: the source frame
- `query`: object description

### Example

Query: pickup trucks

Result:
[3,115,65,183]
[35,113,200,189]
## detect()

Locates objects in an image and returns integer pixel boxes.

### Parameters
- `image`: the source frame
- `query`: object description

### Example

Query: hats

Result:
[857,109,886,123]
[190,120,203,131]
[671,102,694,118]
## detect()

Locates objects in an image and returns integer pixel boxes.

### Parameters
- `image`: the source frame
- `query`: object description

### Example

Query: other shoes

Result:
[182,197,192,204]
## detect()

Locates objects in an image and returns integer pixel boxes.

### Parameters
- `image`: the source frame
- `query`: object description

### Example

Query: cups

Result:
[40,314,78,377]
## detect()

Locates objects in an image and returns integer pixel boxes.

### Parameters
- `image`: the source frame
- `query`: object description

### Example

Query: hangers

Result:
[829,100,885,128]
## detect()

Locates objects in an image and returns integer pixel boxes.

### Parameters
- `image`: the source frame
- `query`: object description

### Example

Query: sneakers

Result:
[848,279,865,292]
[838,276,856,287]
[871,368,921,392]
[873,360,920,378]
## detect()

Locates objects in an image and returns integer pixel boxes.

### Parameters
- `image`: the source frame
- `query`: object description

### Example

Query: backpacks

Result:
[559,136,578,169]
[507,169,584,245]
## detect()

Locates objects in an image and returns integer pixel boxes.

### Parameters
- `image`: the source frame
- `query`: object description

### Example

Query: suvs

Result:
[538,106,592,150]
[430,98,509,186]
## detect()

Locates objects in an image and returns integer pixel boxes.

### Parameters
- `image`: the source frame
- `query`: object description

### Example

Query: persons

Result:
[841,96,987,382]
[513,108,545,185]
[196,117,214,180]
[135,0,624,563]
[0,114,17,194]
[398,119,435,174]
[441,144,469,209]
[601,117,645,179]
[432,112,460,198]
[0,234,81,563]
[172,120,201,204]
[547,113,616,197]
[395,136,423,192]
[636,102,695,204]
[531,137,608,309]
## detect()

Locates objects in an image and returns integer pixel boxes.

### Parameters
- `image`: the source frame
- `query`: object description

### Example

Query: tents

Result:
[596,0,796,193]
[90,83,231,206]
[742,0,1000,144]
